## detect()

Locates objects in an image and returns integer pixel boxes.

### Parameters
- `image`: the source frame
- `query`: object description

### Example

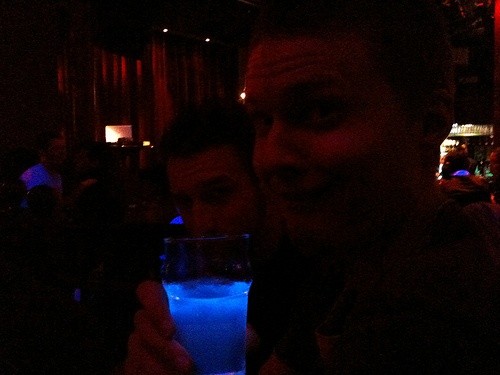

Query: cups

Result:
[164,233,250,375]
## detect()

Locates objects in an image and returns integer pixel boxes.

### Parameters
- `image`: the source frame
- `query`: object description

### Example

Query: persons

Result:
[154,104,314,355]
[475,147,500,204]
[442,136,478,180]
[19,131,96,234]
[69,140,114,222]
[128,0,500,375]
